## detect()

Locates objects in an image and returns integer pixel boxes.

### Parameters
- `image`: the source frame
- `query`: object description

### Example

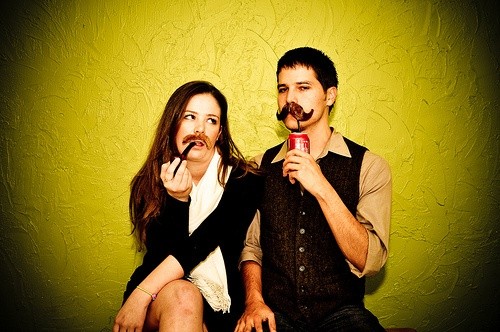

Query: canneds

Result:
[286,132,311,155]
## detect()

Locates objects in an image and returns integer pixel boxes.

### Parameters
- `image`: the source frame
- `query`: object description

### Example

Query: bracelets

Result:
[136,286,156,300]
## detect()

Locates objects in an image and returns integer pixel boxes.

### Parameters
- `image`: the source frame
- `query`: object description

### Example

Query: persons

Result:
[231,43,397,331]
[111,81,257,332]
